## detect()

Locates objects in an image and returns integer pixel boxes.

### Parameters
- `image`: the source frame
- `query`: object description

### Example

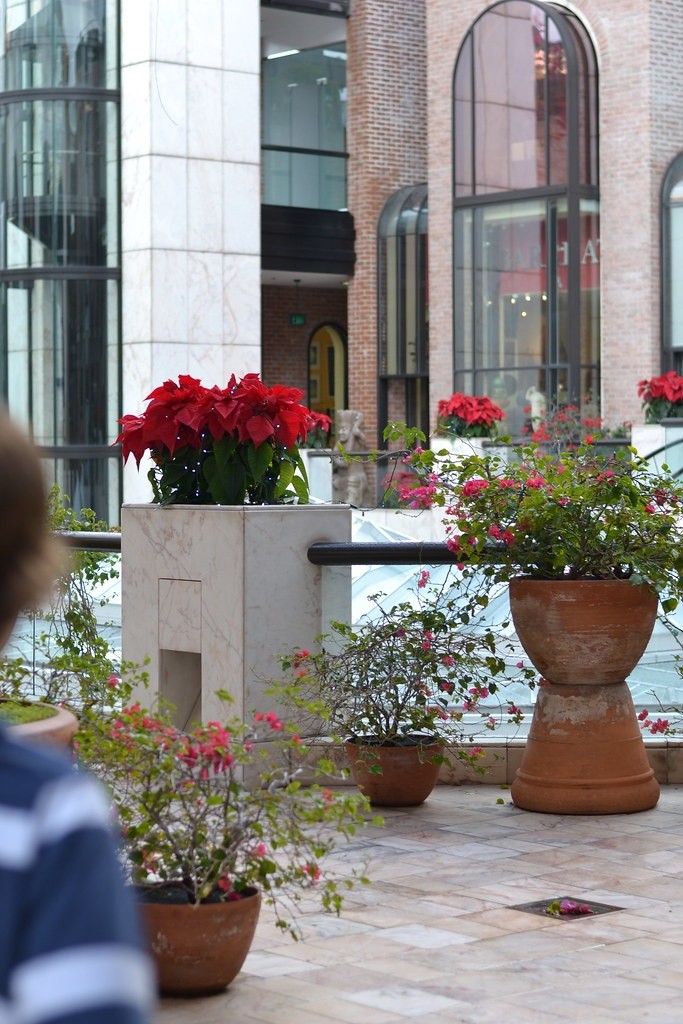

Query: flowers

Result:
[116,372,331,506]
[398,417,683,675]
[268,530,556,784]
[436,392,506,439]
[636,372,683,423]
[65,676,389,942]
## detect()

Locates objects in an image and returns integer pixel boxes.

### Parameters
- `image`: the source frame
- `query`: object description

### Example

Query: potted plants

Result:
[0,654,77,750]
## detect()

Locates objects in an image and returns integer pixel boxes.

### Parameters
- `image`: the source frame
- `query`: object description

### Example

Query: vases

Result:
[344,733,444,806]
[509,578,659,813]
[123,505,354,739]
[630,424,683,490]
[428,436,507,509]
[280,450,333,504]
[130,880,263,997]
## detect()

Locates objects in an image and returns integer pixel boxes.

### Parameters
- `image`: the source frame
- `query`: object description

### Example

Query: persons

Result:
[525,385,545,432]
[331,410,366,509]
[0,397,160,1024]
